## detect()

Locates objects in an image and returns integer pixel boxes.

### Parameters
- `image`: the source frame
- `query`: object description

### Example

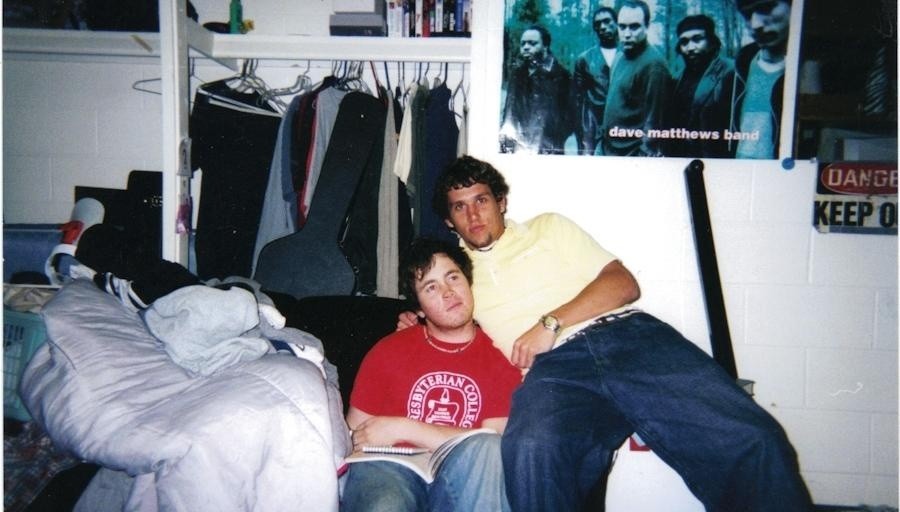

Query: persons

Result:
[602,0,671,155]
[735,0,791,159]
[396,155,814,512]
[500,27,573,154]
[341,239,522,512]
[573,7,618,155]
[671,14,735,158]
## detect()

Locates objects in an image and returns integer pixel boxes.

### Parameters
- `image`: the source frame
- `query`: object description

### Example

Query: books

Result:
[344,427,498,484]
[386,0,471,37]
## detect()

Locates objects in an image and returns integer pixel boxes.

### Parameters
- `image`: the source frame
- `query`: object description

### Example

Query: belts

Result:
[552,304,643,350]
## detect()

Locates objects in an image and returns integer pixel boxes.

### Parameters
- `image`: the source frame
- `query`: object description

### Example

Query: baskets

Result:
[3,305,46,424]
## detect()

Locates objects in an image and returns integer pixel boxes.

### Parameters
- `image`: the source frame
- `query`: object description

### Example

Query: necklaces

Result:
[424,325,476,354]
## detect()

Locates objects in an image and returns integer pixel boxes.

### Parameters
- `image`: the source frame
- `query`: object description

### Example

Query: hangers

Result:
[131,55,205,104]
[196,59,468,119]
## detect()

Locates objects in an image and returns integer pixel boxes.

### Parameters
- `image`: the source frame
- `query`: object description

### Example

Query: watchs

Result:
[539,315,562,333]
